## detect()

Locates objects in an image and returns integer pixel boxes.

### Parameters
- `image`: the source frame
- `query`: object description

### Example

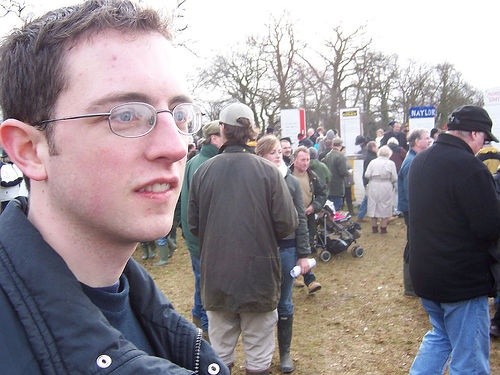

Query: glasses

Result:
[478,132,490,145]
[31,102,206,139]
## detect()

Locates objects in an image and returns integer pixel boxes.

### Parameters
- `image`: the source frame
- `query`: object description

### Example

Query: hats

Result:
[448,104,500,143]
[332,138,343,144]
[202,120,219,138]
[219,102,255,128]
[1,150,8,158]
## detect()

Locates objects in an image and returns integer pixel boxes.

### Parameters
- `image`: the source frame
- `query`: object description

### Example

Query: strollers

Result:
[313,199,364,263]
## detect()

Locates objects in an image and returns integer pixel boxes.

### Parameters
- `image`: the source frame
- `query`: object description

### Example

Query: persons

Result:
[0,150,29,214]
[279,127,354,294]
[408,106,500,375]
[141,123,204,265]
[187,102,300,375]
[254,134,313,374]
[0,0,231,375]
[354,121,445,234]
[166,120,223,344]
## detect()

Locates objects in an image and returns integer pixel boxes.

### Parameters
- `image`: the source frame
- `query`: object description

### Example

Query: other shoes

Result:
[381,227,387,234]
[404,289,418,298]
[306,280,321,295]
[349,210,353,215]
[393,214,402,218]
[490,311,500,340]
[372,226,378,233]
[356,218,367,223]
[293,275,305,287]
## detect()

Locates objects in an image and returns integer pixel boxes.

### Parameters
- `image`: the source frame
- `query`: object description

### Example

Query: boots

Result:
[151,245,168,266]
[166,237,178,258]
[149,245,156,259]
[277,315,295,372]
[246,368,268,375]
[192,315,209,342]
[142,247,148,260]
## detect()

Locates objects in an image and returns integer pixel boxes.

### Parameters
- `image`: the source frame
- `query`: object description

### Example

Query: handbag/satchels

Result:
[0,162,24,187]
